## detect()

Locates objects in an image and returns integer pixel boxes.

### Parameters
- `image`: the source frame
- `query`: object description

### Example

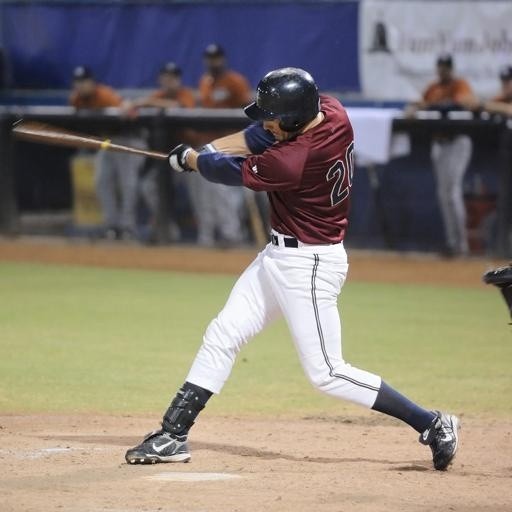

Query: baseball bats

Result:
[13,123,169,161]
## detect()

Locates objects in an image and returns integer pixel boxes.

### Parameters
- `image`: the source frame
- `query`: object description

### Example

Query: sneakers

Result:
[125,429,191,464]
[421,411,459,469]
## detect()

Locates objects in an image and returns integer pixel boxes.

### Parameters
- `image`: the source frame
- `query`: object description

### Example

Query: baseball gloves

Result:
[483,263,512,316]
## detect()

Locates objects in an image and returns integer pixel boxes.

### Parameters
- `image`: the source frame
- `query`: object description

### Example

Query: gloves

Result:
[168,143,218,173]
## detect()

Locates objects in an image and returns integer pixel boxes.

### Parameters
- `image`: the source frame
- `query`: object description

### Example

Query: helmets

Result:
[243,67,320,131]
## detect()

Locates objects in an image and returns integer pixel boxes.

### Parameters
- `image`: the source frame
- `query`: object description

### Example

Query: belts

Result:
[269,232,300,248]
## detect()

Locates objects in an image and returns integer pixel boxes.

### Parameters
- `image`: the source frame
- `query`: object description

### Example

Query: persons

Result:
[128,62,215,246]
[404,53,480,258]
[124,66,459,471]
[482,65,512,117]
[68,66,148,242]
[201,45,272,241]
